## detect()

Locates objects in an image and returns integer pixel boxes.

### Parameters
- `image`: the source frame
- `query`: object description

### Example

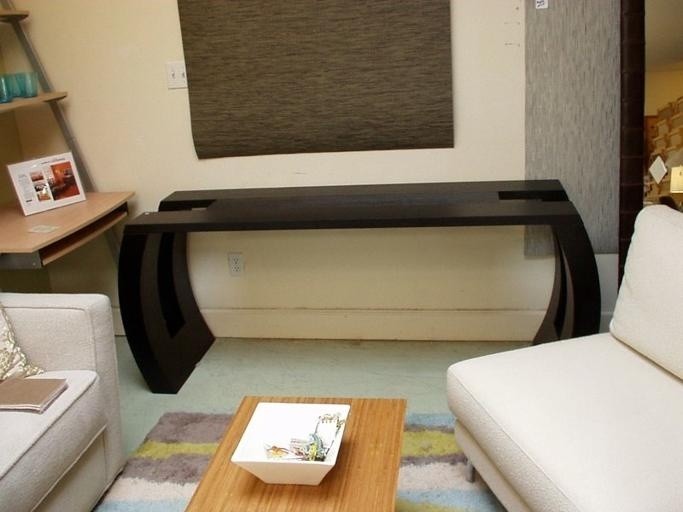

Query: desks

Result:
[115,176,603,394]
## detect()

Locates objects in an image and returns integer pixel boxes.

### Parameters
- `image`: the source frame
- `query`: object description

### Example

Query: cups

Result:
[0,72,39,103]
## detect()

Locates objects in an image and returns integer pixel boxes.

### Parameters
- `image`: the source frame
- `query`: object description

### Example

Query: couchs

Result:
[446,200,683,512]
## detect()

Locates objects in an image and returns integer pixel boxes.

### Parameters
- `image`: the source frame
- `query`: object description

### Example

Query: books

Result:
[0,375,70,415]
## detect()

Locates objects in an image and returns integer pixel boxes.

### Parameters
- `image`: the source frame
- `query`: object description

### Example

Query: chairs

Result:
[0,289,130,512]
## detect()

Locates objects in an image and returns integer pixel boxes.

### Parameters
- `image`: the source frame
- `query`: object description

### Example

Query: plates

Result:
[230,401,350,486]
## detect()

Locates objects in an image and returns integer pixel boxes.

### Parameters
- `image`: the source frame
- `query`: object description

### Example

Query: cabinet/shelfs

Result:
[0,0,130,278]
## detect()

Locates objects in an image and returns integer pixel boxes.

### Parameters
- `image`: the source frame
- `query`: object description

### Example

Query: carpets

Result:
[87,409,503,512]
